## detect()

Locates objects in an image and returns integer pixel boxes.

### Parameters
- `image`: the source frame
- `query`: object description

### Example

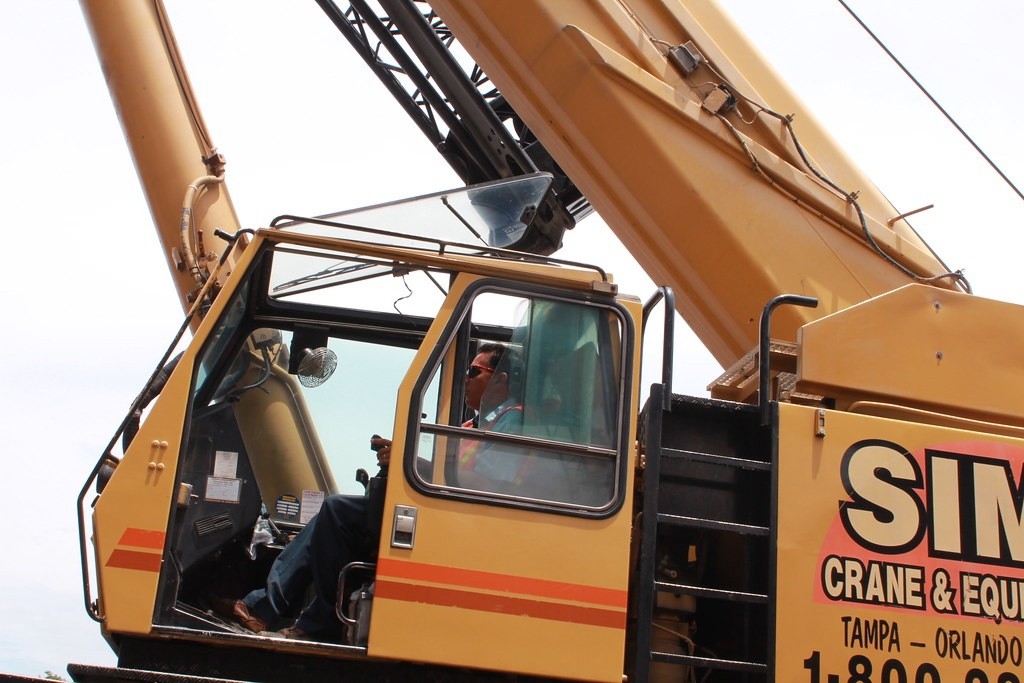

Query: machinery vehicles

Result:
[0,1,1023,683]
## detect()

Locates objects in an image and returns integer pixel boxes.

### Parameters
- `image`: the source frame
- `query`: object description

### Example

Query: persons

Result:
[230,341,524,641]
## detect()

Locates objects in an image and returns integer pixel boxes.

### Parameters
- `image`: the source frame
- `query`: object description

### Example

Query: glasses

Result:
[467,365,494,378]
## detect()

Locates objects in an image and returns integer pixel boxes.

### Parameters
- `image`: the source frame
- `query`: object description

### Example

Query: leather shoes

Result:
[256,626,316,641]
[209,597,269,632]
